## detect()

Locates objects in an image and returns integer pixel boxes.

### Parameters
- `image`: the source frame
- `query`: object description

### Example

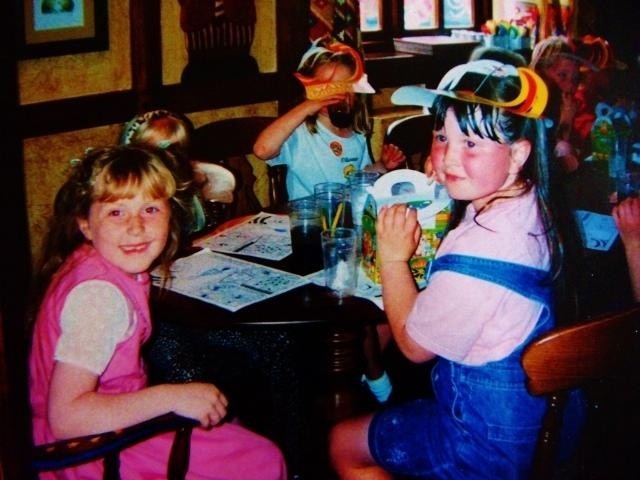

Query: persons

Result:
[613,196,640,304]
[252,44,407,201]
[25,142,290,479]
[327,54,591,480]
[118,107,235,235]
[529,36,584,139]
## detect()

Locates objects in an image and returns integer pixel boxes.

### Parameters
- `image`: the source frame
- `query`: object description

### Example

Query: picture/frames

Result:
[15,1,111,62]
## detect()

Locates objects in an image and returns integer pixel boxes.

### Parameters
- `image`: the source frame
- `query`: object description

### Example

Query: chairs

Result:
[23,409,216,480]
[184,116,281,225]
[520,303,640,478]
[383,112,436,172]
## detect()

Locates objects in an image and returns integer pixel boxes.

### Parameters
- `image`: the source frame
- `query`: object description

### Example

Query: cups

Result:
[607,138,640,205]
[328,96,354,129]
[319,227,360,300]
[288,171,380,264]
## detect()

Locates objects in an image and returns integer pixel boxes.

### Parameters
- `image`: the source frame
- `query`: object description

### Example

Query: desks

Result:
[147,194,389,421]
